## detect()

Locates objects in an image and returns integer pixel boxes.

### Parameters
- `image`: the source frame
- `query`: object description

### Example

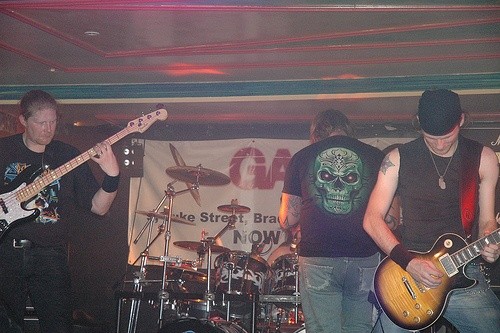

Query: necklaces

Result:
[426,137,460,189]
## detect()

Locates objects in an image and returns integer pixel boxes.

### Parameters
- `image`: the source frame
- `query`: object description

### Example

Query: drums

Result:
[213,249,271,319]
[184,299,215,318]
[270,253,303,310]
[167,260,202,267]
[158,315,249,333]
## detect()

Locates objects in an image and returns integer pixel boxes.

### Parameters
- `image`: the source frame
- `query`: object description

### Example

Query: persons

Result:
[0,90,122,333]
[363,89,500,333]
[268,109,408,333]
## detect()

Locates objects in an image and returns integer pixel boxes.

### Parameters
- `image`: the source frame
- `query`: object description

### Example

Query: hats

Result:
[418,89,462,136]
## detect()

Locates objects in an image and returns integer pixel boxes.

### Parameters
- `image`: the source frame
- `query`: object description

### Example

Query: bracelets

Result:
[389,244,415,272]
[101,175,120,192]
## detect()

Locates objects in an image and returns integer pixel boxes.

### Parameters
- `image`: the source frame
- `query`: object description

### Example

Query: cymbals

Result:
[164,166,232,186]
[172,240,231,253]
[217,205,250,212]
[169,143,201,207]
[133,209,197,226]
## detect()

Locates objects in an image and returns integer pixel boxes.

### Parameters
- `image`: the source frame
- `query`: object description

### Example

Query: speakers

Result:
[119,145,144,177]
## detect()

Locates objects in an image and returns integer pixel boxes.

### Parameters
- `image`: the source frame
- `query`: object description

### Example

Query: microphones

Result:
[258,236,268,254]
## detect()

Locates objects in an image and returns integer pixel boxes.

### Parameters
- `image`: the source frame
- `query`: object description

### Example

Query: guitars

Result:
[373,227,500,333]
[1,102,169,242]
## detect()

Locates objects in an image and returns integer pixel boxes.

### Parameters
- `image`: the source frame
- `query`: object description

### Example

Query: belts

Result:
[10,238,34,250]
